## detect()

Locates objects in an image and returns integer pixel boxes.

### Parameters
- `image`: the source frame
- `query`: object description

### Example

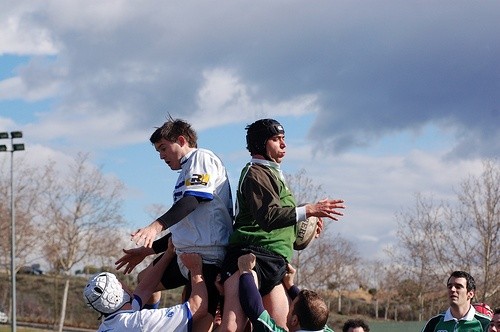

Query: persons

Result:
[114,119,233,332]
[419,270,500,332]
[238,253,335,332]
[214,118,346,332]
[342,319,371,332]
[82,235,208,332]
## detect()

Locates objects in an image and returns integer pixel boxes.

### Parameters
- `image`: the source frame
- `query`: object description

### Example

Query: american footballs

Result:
[293,202,320,251]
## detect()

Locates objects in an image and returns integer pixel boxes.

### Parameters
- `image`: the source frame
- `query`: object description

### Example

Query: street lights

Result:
[0,131,26,332]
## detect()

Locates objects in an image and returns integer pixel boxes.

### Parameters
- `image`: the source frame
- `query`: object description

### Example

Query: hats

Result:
[83,272,130,315]
[246,118,285,155]
